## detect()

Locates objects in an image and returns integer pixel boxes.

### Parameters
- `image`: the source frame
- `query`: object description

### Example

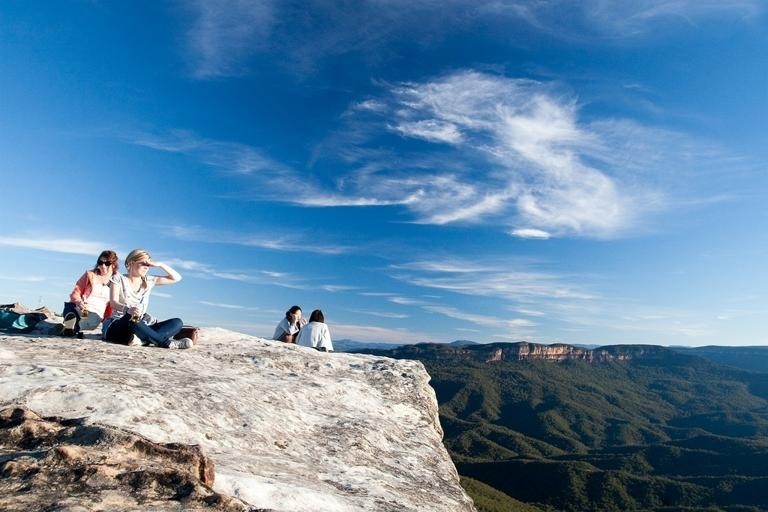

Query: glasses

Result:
[98,260,113,266]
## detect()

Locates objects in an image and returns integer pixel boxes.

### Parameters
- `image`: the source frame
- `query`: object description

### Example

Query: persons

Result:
[274,306,308,343]
[295,309,333,352]
[60,250,124,337]
[103,249,194,350]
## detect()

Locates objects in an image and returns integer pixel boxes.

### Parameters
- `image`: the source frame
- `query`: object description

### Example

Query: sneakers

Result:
[169,337,193,349]
[128,332,146,346]
[55,312,77,337]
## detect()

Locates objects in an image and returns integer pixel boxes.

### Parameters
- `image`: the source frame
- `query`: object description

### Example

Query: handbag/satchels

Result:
[173,327,198,344]
[0,309,47,334]
